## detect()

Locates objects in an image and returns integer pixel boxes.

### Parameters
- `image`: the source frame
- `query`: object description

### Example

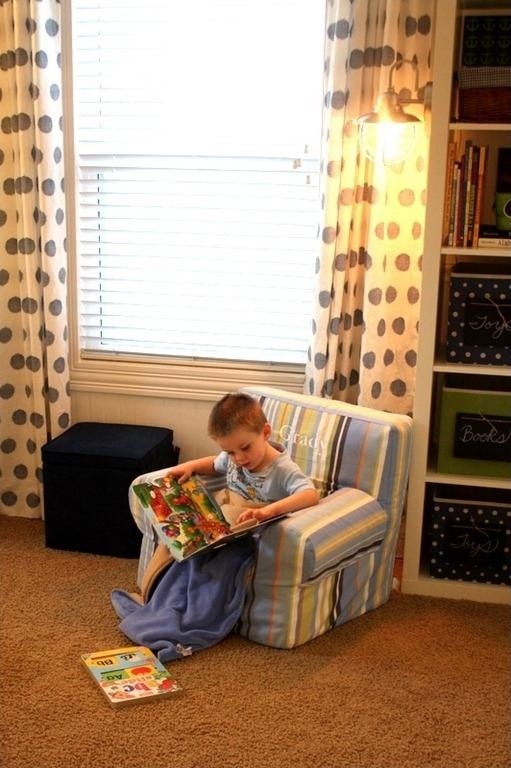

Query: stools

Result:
[41,421,179,558]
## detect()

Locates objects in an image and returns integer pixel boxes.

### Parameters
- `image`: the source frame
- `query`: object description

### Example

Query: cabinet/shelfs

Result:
[400,0,511,604]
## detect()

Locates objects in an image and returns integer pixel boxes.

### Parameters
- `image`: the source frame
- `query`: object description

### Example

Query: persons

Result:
[128,391,323,628]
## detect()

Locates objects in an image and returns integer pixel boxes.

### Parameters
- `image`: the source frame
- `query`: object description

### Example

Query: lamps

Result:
[352,60,425,165]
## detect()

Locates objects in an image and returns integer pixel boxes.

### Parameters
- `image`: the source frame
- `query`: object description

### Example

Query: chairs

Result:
[128,386,413,648]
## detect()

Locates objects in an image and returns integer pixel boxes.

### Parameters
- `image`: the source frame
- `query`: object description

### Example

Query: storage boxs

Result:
[430,491,511,586]
[437,383,511,475]
[446,262,511,366]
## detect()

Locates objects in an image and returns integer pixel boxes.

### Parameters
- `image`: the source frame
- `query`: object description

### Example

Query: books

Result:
[440,136,511,253]
[131,473,292,567]
[80,645,186,711]
